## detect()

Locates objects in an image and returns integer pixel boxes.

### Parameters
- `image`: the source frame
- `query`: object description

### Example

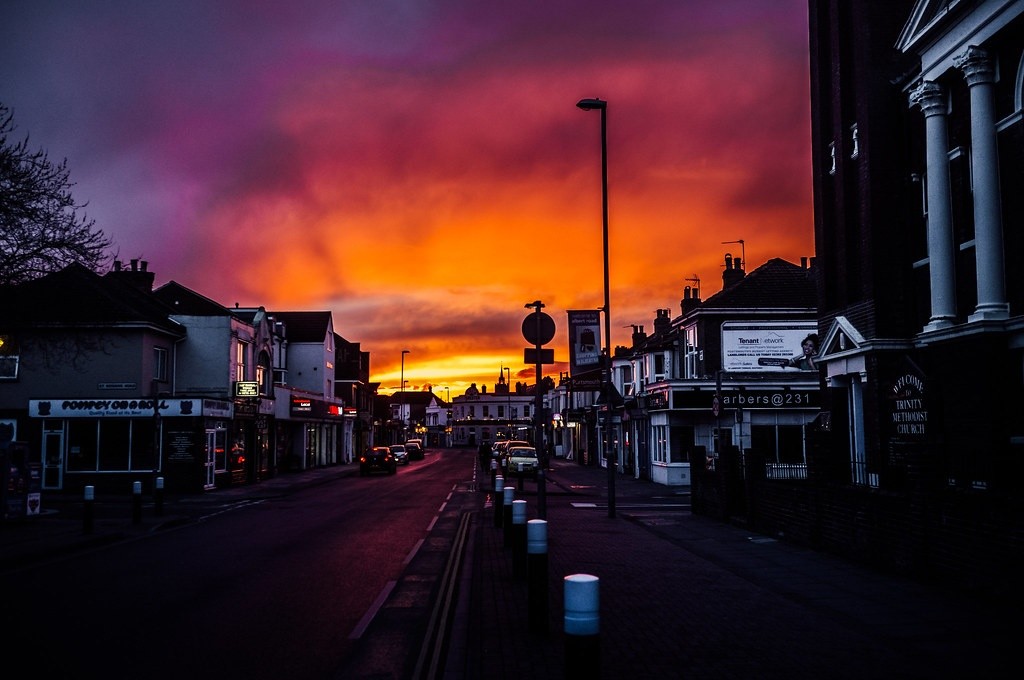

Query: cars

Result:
[406,438,425,459]
[390,444,408,464]
[491,442,540,474]
[360,448,396,474]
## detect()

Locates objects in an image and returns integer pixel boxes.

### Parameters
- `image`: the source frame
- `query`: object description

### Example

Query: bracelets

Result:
[792,358,796,364]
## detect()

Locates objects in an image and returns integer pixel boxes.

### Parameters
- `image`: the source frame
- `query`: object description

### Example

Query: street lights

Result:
[525,301,545,521]
[404,380,408,425]
[504,368,511,438]
[577,97,616,521]
[152,378,169,511]
[401,350,410,444]
[445,387,450,448]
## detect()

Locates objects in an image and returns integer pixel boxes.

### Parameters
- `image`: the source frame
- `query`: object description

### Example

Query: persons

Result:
[787,333,819,370]
